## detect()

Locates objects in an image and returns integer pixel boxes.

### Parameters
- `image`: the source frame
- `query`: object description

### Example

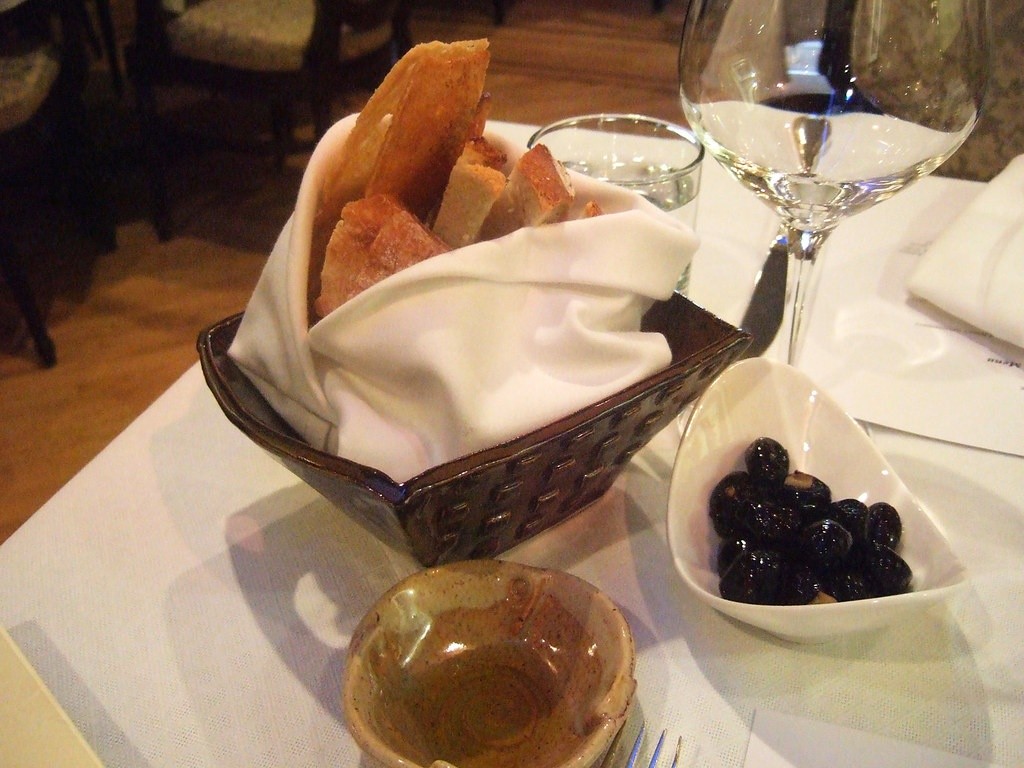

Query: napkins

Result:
[908,154,1024,347]
[743,708,1001,768]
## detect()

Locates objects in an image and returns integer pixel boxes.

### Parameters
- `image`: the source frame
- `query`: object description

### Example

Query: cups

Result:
[526,113,705,301]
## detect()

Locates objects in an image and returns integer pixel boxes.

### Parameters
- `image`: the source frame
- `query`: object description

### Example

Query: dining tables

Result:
[0,113,1024,768]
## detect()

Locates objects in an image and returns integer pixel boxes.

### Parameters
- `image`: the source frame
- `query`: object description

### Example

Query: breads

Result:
[310,43,603,326]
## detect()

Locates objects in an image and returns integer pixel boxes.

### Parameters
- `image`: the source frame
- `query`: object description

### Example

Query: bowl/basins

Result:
[666,356,976,644]
[340,559,634,768]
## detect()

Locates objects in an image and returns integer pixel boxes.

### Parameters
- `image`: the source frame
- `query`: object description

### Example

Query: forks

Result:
[599,716,683,768]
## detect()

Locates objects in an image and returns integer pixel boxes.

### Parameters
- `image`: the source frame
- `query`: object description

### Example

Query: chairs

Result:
[0,0,415,368]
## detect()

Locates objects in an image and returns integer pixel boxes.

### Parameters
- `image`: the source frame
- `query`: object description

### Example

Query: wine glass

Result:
[677,0,993,366]
